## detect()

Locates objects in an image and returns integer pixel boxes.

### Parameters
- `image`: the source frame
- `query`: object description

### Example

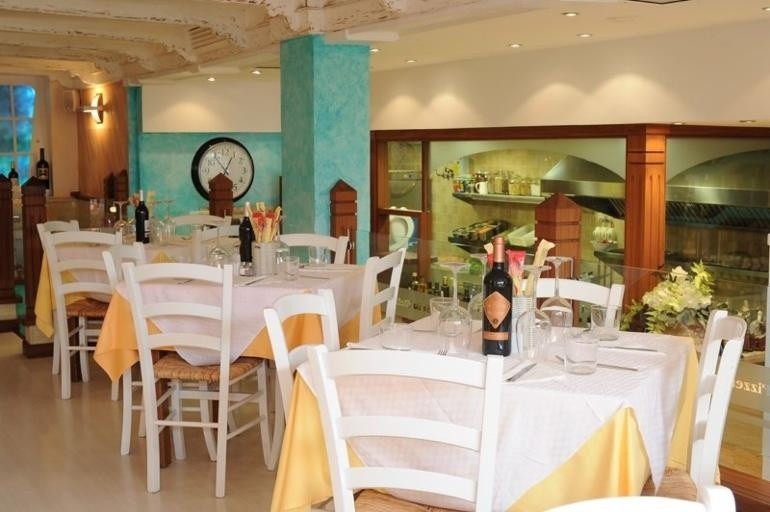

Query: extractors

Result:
[544,152,769,229]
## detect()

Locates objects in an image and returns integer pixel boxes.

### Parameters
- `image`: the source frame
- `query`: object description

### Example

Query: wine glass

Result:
[514,265,553,362]
[113,198,137,245]
[206,218,230,272]
[270,214,290,255]
[149,198,177,246]
[435,262,473,354]
[545,257,586,346]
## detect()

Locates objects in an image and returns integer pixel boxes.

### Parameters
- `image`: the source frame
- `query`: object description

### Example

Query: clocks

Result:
[190,136,255,204]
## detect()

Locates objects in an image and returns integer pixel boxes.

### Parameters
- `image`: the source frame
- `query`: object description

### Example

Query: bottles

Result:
[718,294,765,340]
[8,160,19,187]
[37,148,50,188]
[236,201,256,276]
[409,271,474,302]
[136,188,150,245]
[453,170,538,197]
[481,238,514,355]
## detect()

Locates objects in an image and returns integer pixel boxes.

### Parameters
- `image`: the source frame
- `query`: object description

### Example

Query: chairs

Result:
[597,307,747,474]
[304,343,506,512]
[545,484,737,512]
[514,276,627,333]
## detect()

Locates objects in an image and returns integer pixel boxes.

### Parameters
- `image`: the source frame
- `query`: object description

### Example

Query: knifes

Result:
[507,361,536,384]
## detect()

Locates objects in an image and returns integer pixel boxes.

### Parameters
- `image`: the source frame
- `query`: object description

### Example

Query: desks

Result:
[405,252,438,289]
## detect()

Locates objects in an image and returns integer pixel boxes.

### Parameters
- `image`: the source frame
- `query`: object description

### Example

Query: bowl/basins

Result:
[590,240,617,253]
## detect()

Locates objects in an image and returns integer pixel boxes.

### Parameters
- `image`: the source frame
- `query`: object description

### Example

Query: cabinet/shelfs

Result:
[446,191,551,260]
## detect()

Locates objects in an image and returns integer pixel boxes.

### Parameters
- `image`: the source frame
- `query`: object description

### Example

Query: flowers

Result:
[622,259,716,336]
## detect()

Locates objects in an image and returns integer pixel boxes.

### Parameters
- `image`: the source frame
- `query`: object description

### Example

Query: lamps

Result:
[78,93,106,124]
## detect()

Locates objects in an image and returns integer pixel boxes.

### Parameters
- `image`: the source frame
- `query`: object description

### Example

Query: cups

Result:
[512,296,534,352]
[276,254,300,279]
[250,239,280,275]
[565,327,602,373]
[308,241,330,270]
[429,298,459,330]
[590,304,620,342]
[378,322,414,351]
